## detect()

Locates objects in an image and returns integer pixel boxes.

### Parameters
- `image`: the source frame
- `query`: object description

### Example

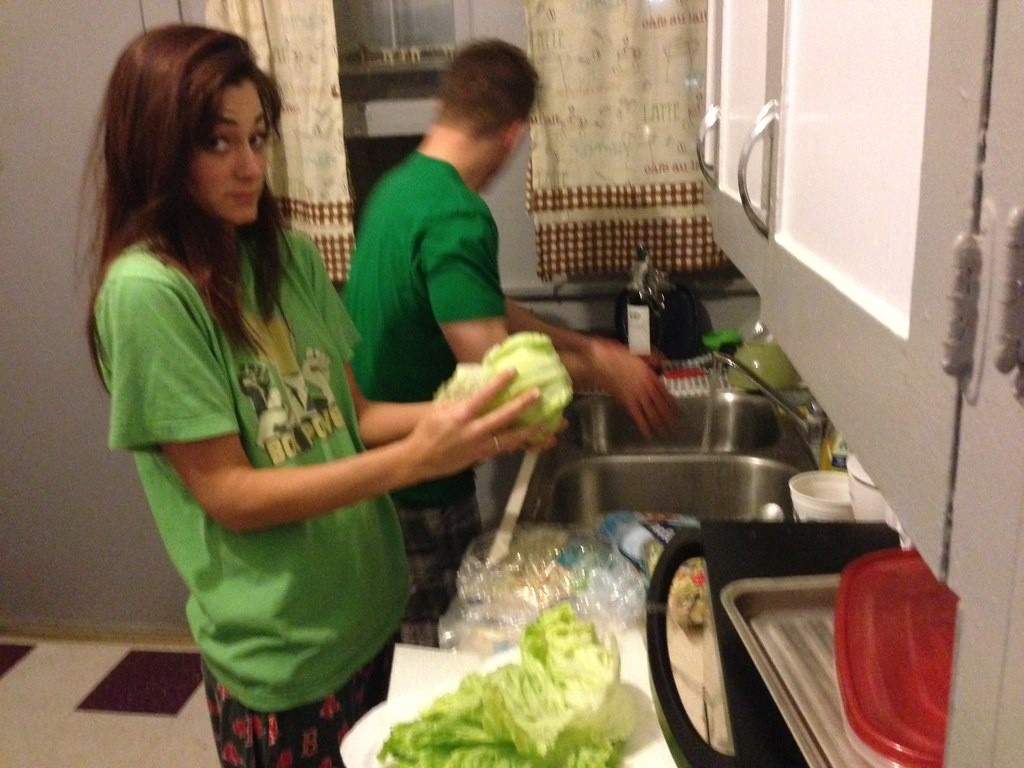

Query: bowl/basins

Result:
[789,470,856,523]
[728,340,796,392]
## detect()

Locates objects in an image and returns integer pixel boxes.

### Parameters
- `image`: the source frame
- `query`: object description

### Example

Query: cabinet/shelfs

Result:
[697,2,1024,768]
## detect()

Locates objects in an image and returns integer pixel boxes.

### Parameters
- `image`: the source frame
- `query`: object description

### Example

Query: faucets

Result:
[710,348,825,445]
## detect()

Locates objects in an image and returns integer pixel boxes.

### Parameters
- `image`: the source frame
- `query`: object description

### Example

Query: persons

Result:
[85,23,572,768]
[336,37,688,642]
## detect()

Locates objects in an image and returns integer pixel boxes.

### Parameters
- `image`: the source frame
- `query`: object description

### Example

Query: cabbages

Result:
[433,331,572,445]
[373,600,637,767]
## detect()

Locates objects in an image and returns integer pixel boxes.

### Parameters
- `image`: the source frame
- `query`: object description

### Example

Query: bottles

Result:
[614,244,663,357]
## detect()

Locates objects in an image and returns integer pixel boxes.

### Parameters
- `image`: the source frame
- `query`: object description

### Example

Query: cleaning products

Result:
[614,244,665,363]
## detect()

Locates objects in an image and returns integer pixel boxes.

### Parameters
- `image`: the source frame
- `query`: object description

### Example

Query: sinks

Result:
[516,458,819,527]
[524,394,821,457]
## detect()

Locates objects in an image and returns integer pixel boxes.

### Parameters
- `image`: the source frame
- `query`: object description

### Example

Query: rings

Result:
[493,435,502,456]
